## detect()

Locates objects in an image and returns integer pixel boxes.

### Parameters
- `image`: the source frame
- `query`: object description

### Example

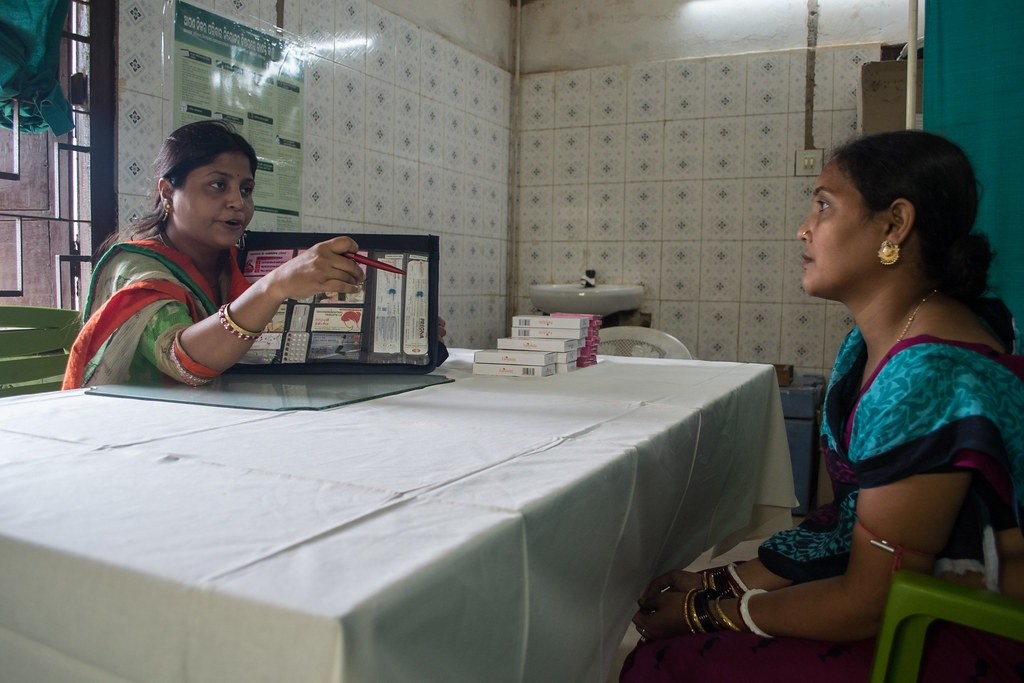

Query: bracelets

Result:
[684,561,777,641]
[218,302,264,343]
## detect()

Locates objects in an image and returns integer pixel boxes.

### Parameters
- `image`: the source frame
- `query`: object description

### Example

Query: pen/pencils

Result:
[341,248,408,279]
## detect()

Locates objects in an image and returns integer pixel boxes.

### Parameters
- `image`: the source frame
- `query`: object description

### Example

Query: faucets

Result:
[580,269,596,288]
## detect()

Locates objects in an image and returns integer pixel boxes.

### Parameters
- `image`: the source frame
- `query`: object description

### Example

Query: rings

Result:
[640,629,647,642]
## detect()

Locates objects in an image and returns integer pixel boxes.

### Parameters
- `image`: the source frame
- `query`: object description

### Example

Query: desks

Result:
[0,347,800,683]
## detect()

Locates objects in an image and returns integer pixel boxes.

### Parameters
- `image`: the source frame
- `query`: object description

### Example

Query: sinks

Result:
[528,283,645,318]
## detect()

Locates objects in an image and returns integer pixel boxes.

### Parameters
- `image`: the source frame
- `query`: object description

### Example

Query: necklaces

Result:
[895,289,935,344]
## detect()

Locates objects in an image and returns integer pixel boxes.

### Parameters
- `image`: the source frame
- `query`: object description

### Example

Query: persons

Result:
[617,129,1024,682]
[59,121,366,389]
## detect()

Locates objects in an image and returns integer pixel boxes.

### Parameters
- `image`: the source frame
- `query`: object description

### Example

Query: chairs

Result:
[0,304,86,399]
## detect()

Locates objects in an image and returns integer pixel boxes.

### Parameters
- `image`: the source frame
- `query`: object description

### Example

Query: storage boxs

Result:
[472,313,603,376]
